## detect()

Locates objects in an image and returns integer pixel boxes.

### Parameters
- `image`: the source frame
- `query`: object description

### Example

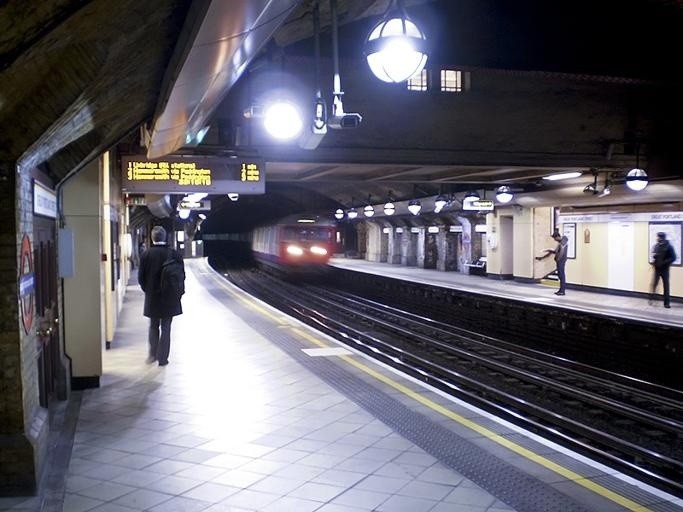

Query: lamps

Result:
[362,1,431,85]
[332,180,515,222]
[623,130,647,193]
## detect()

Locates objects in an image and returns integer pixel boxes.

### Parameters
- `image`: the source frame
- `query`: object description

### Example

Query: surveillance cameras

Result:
[327,113,363,129]
[297,119,327,150]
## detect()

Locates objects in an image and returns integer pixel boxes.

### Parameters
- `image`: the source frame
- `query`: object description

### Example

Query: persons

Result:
[647,231,676,309]
[548,232,568,295]
[136,224,187,366]
[138,241,145,253]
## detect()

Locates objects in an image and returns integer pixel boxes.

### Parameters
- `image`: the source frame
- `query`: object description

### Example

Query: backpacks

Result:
[160,248,185,297]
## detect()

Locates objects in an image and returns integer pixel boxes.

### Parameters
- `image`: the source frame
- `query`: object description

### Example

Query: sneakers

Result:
[664,304,671,308]
[150,347,169,366]
[554,291,565,295]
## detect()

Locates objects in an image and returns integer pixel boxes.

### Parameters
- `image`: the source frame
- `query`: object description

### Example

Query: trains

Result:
[250,212,338,281]
